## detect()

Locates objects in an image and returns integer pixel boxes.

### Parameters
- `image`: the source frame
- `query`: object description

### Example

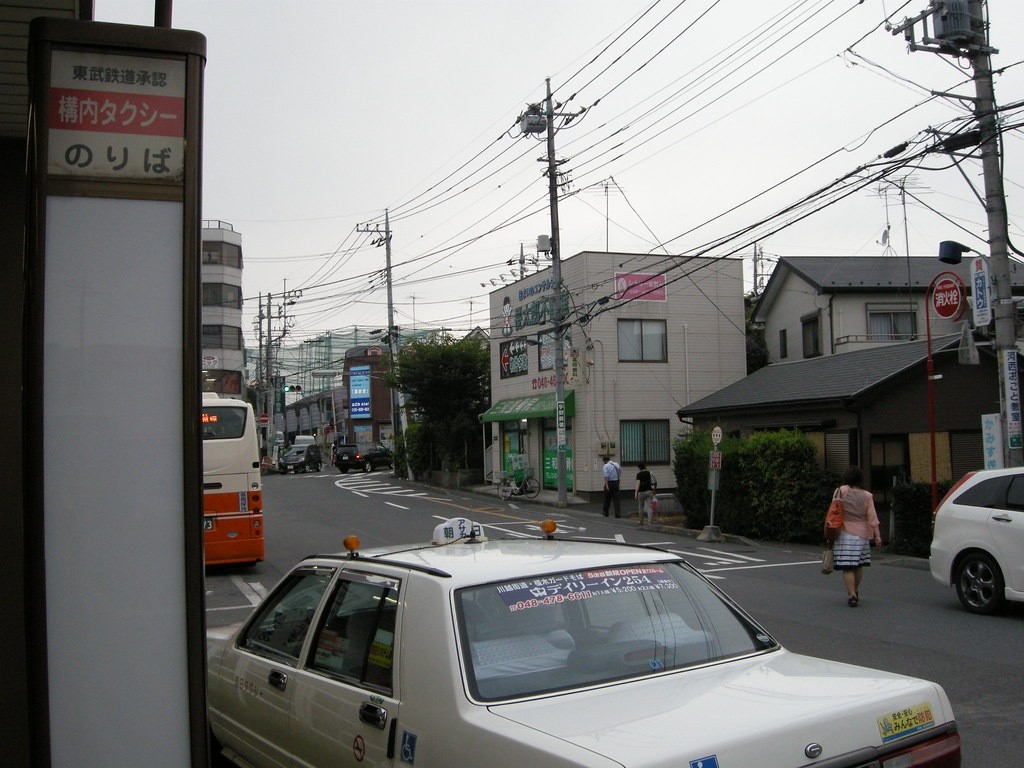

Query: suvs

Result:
[334,441,395,475]
[277,442,323,475]
[927,465,1024,615]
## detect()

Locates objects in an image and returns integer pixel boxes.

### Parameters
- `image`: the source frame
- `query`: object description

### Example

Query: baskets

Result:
[525,468,535,477]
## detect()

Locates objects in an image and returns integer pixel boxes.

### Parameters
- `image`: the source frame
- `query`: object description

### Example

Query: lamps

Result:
[480,259,552,288]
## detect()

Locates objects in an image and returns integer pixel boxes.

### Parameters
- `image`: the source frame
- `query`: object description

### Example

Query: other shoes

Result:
[601,511,609,516]
[615,515,620,518]
[848,592,859,607]
[638,521,643,525]
[648,522,652,525]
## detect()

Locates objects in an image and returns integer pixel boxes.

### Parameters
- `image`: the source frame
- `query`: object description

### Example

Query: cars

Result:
[206,516,962,768]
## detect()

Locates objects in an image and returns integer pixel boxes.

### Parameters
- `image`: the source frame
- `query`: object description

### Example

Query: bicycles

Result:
[498,465,541,502]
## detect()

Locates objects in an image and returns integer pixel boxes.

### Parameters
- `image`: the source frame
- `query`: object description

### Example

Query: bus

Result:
[202,390,267,573]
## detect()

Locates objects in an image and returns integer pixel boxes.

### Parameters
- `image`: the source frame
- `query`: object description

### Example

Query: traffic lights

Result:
[282,385,304,393]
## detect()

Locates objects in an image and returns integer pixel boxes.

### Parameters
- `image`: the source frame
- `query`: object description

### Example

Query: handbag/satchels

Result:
[824,487,843,543]
[821,543,834,574]
[647,470,655,484]
[644,496,657,513]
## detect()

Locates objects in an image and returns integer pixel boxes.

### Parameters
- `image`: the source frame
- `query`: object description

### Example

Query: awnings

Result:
[482,389,575,423]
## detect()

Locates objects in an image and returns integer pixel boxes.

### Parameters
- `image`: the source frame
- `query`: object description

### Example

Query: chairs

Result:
[339,609,396,678]
[470,629,578,707]
[598,613,714,680]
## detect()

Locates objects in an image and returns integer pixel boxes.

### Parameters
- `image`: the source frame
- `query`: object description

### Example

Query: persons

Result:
[635,462,657,524]
[601,456,621,518]
[827,466,882,608]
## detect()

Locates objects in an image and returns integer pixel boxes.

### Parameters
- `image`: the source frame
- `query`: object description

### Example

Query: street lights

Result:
[525,337,568,508]
[936,240,1024,468]
[370,325,401,475]
